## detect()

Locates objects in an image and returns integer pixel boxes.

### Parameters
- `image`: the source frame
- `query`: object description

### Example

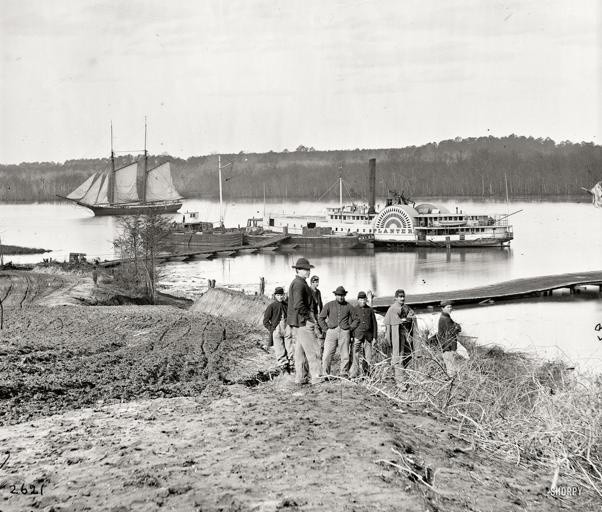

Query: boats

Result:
[132,209,376,255]
[324,156,516,250]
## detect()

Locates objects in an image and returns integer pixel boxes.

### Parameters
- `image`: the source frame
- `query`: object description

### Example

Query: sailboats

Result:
[55,116,184,217]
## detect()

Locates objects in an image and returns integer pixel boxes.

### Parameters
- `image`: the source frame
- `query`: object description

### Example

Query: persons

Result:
[262,257,377,385]
[438,299,461,377]
[384,289,417,391]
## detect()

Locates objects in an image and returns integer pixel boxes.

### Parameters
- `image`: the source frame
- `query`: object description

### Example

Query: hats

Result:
[358,291,366,298]
[292,258,314,269]
[332,286,348,294]
[438,299,455,307]
[274,287,284,294]
